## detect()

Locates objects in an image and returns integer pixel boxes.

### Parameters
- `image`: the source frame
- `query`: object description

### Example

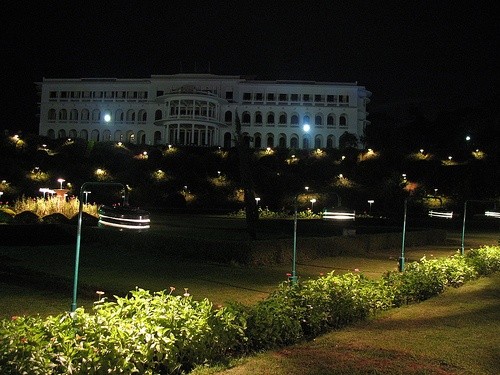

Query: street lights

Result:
[367,200,375,217]
[255,197,261,206]
[83,191,91,207]
[39,187,50,199]
[56,178,66,190]
[310,199,316,212]
